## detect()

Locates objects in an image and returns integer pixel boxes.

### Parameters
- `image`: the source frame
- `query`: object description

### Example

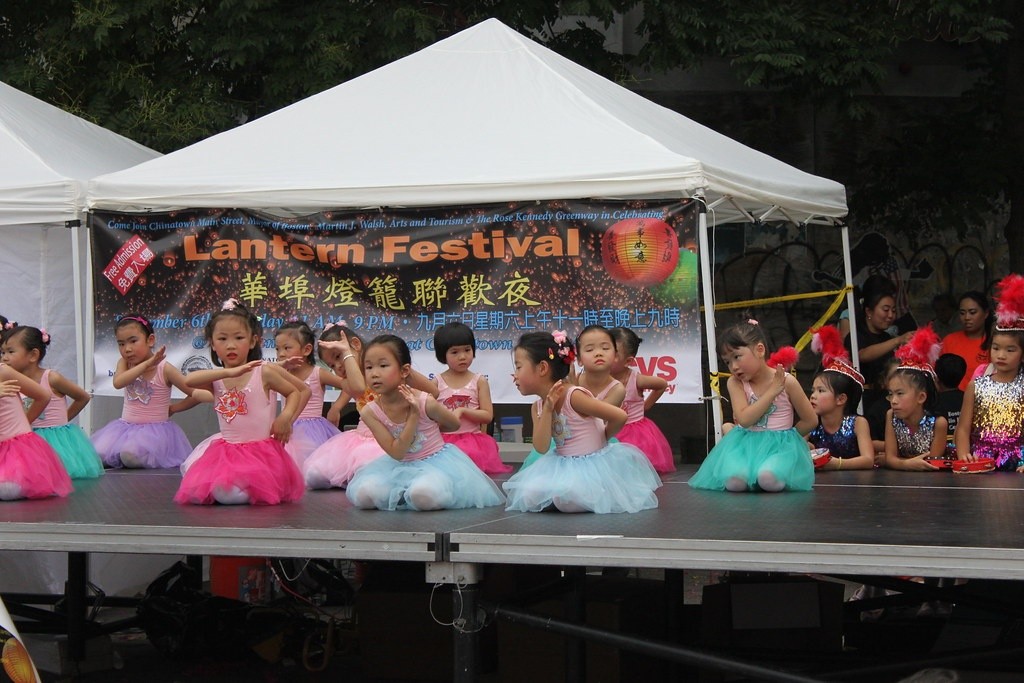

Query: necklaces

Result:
[623,368,629,384]
[380,397,408,423]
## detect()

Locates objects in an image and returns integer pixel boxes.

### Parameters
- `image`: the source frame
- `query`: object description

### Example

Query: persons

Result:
[0,315,105,477]
[173,297,312,504]
[275,320,343,467]
[432,323,514,473]
[303,321,441,490]
[803,370,874,470]
[90,314,202,468]
[347,334,507,509]
[502,331,677,513]
[838,274,992,441]
[0,362,75,501]
[686,318,817,491]
[884,370,947,470]
[956,330,1024,472]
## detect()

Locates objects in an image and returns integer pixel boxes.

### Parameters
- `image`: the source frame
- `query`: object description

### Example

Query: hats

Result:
[808,325,867,392]
[992,275,1024,331]
[896,326,943,391]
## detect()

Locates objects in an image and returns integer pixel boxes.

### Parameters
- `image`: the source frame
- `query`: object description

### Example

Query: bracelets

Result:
[343,354,355,362]
[837,457,841,469]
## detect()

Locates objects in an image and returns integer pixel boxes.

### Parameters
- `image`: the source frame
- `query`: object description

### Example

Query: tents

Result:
[83,18,864,448]
[0,80,165,427]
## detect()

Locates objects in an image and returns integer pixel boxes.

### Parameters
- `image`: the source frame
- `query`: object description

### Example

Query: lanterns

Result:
[602,217,696,311]
